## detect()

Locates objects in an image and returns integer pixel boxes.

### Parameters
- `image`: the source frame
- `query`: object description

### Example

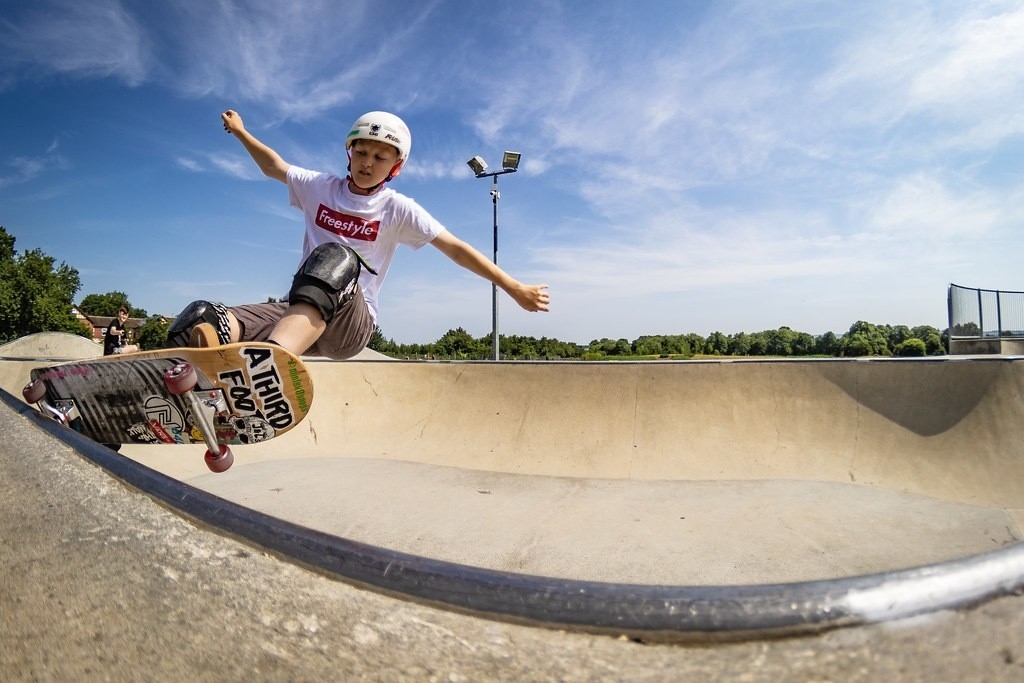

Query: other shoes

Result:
[187,323,224,348]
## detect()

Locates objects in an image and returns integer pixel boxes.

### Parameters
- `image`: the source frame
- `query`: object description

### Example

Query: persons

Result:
[166,108,554,361]
[104,306,139,357]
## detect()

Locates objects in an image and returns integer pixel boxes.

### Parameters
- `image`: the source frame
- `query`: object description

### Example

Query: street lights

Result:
[465,150,521,361]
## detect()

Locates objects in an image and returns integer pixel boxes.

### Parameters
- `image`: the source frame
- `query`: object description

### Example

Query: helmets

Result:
[345,112,411,168]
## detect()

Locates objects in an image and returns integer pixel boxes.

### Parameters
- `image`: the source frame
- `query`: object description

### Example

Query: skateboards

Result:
[21,341,315,473]
